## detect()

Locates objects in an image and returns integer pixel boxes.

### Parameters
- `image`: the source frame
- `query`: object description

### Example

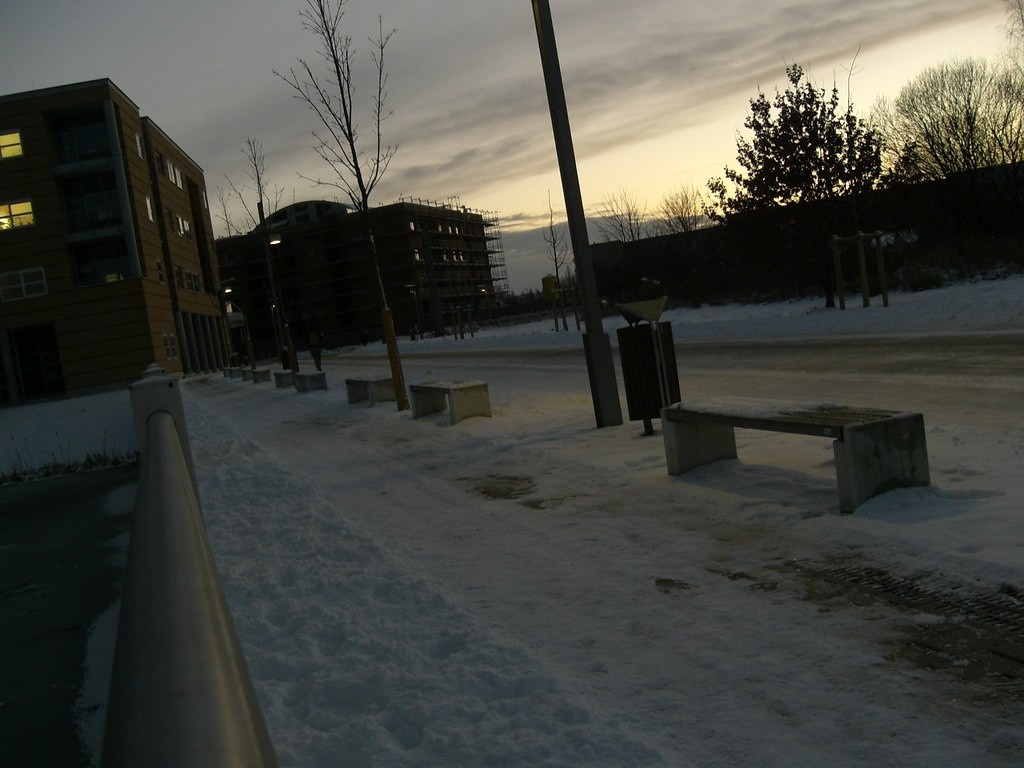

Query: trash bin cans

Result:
[616,296,682,434]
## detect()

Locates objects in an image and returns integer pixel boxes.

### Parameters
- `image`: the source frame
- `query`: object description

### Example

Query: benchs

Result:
[345,373,395,406]
[224,366,271,384]
[410,378,492,425]
[661,395,929,513]
[274,370,293,387]
[295,372,327,391]
